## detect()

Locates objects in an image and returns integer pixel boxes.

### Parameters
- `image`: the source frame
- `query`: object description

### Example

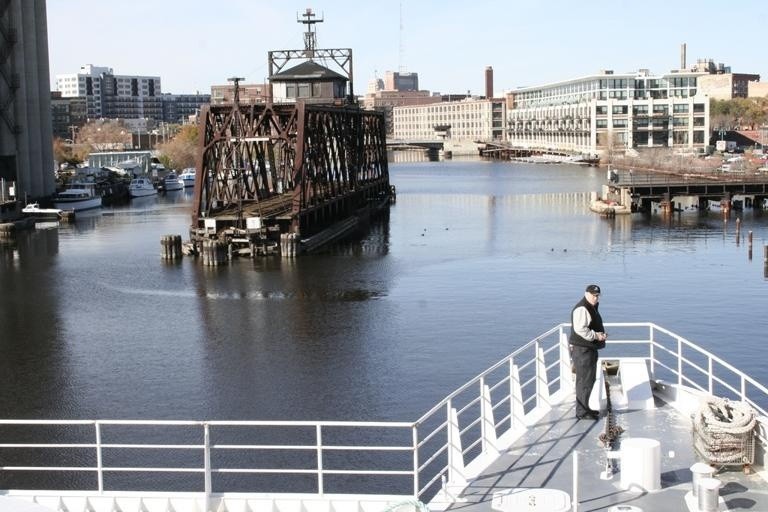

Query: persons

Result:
[566,283,608,421]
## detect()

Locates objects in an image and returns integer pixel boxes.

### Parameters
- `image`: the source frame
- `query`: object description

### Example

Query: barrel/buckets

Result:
[692,463,711,496]
[698,477,722,511]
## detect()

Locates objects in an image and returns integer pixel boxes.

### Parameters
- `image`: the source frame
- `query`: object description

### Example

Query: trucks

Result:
[721,157,744,172]
[716,140,738,152]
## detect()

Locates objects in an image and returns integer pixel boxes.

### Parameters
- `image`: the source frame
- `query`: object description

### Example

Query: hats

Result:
[585,285,600,295]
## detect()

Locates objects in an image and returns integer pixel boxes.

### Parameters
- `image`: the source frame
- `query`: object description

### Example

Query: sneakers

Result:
[586,409,599,415]
[577,413,599,421]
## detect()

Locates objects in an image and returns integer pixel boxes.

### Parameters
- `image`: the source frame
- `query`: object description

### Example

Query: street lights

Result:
[718,128,728,141]
[65,137,75,158]
[147,121,177,148]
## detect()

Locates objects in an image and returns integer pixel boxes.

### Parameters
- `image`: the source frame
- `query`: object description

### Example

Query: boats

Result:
[19,160,270,224]
